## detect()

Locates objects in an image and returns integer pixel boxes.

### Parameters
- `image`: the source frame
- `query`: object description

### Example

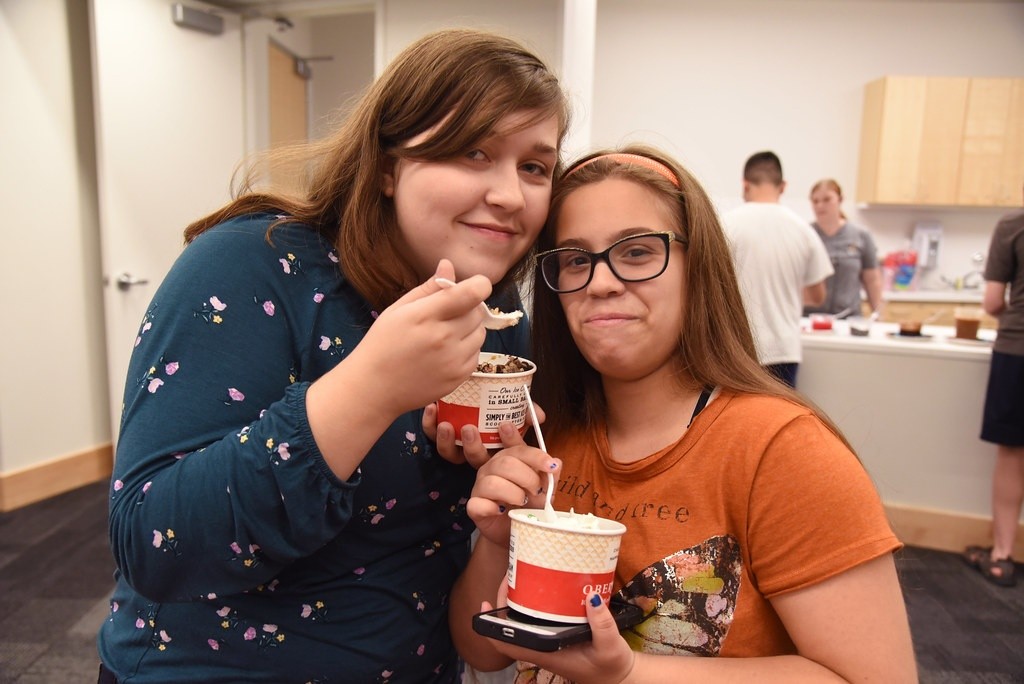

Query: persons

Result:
[964,211,1024,587]
[451,144,919,684]
[98,31,571,684]
[801,180,884,323]
[717,151,835,389]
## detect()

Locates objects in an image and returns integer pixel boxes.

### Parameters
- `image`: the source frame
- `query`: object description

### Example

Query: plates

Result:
[948,336,993,347]
[887,333,932,341]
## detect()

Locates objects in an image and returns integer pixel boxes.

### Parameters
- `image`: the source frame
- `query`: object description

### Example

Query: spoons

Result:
[436,278,522,329]
[523,386,558,523]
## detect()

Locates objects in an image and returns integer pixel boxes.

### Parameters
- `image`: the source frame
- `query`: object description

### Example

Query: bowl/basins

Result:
[507,508,627,625]
[437,352,536,449]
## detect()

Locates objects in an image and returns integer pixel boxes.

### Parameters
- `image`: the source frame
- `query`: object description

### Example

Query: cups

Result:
[955,306,981,339]
[901,320,921,337]
[810,312,835,333]
[848,316,872,337]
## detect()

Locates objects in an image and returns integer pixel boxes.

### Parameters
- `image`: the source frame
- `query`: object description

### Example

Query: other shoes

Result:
[965,545,1016,586]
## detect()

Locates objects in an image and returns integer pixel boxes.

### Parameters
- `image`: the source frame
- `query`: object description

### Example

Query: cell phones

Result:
[472,605,644,652]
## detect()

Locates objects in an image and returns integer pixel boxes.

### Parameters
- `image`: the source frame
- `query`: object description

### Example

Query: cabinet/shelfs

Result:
[852,77,1024,208]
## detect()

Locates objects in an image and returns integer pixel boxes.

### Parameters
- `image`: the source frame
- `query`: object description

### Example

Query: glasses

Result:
[533,230,689,293]
